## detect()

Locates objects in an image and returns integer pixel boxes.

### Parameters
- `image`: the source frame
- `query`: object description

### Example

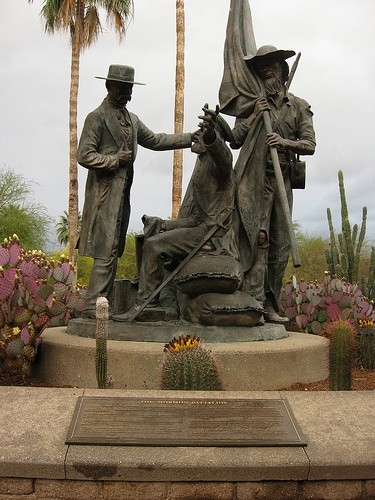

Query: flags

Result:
[219,0,262,116]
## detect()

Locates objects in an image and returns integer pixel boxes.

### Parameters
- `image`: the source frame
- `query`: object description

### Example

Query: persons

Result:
[112,103,233,322]
[230,45,316,324]
[76,65,197,319]
